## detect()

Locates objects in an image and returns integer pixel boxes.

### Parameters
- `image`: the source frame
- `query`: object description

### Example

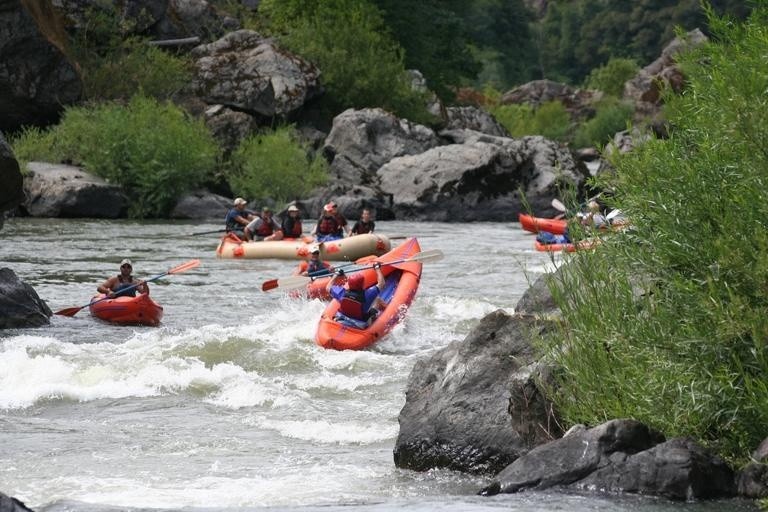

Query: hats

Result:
[121,258,132,268]
[308,245,320,254]
[233,197,248,207]
[323,204,334,211]
[288,205,299,211]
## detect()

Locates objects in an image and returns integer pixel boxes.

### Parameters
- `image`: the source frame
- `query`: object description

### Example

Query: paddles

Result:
[177,225,245,237]
[551,199,575,216]
[599,209,622,225]
[262,254,378,292]
[277,250,445,289]
[53,259,199,317]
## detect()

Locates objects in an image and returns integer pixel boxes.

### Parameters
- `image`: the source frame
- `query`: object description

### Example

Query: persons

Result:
[563,186,618,244]
[280,204,303,237]
[224,197,254,241]
[242,205,281,243]
[324,260,388,323]
[300,245,336,276]
[314,204,340,241]
[309,200,351,235]
[344,209,375,237]
[97,259,150,298]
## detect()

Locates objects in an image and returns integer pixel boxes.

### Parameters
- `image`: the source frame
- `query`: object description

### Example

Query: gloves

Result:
[335,268,345,276]
[372,262,381,270]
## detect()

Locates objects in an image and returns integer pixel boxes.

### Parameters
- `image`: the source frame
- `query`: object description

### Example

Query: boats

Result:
[89,292,164,328]
[289,236,423,352]
[215,231,392,260]
[518,211,633,253]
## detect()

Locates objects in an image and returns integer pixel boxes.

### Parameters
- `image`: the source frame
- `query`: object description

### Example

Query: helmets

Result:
[348,273,364,292]
